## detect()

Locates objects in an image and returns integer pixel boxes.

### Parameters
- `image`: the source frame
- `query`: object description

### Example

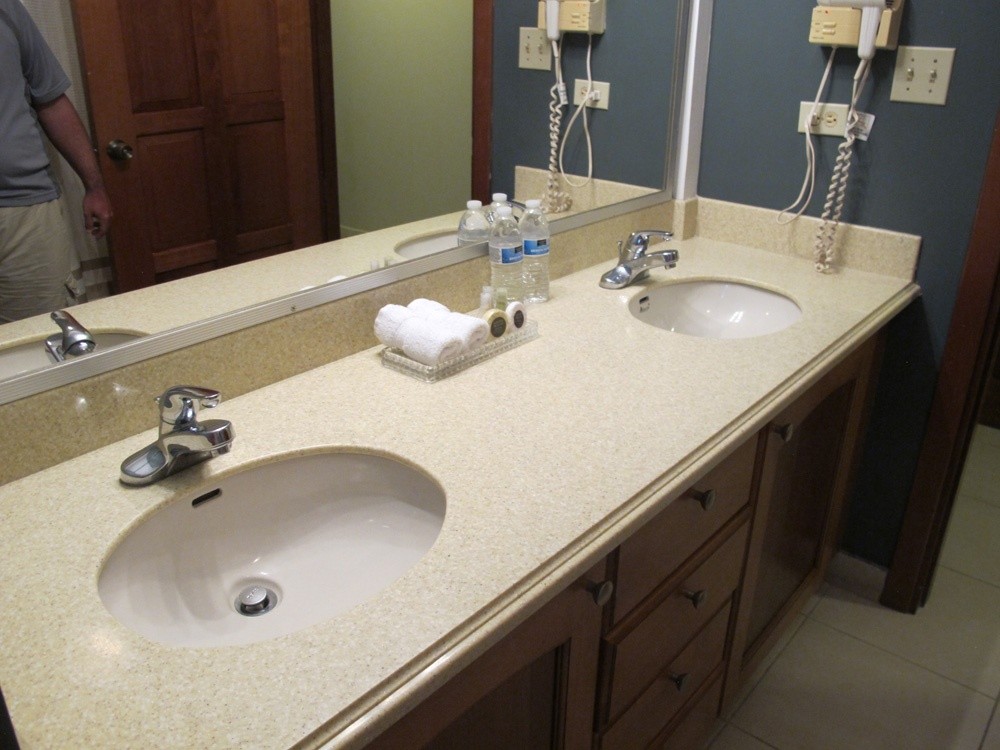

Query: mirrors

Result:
[0,0,693,405]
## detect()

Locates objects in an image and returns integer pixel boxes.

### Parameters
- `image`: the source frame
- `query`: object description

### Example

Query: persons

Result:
[0,0,115,325]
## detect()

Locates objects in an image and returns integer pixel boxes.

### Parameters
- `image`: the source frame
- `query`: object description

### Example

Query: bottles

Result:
[486,192,513,225]
[488,206,524,309]
[518,199,550,302]
[457,199,489,247]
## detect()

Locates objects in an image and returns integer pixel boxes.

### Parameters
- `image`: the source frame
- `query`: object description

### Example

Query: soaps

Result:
[506,302,529,332]
[483,309,509,341]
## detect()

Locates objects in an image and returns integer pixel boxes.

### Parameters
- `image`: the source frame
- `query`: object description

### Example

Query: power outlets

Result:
[798,101,849,136]
[573,79,611,111]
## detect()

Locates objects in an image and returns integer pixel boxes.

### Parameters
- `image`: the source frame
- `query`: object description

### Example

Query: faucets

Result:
[118,384,237,487]
[44,309,96,363]
[487,200,539,227]
[599,228,682,290]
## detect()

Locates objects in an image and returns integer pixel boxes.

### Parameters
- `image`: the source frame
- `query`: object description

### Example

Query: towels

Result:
[406,299,491,351]
[373,303,464,366]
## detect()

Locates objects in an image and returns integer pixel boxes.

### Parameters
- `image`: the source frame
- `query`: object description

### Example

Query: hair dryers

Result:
[816,0,888,61]
[546,0,564,41]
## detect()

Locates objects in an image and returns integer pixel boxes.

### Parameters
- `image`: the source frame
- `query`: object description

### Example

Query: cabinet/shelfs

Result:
[362,332,880,750]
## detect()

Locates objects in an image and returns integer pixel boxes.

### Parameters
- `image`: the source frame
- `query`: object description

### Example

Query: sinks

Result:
[626,274,806,339]
[1,327,152,382]
[392,228,493,259]
[95,441,451,654]
